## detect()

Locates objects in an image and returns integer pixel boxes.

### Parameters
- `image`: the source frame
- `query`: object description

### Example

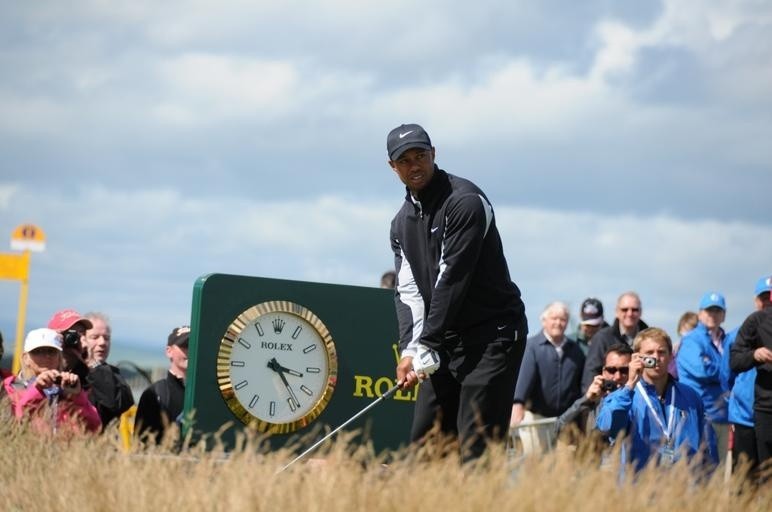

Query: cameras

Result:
[640,357,657,368]
[601,379,618,391]
[63,329,82,350]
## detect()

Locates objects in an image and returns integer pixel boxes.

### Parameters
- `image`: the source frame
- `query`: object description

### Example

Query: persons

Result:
[381,271,397,288]
[0,310,135,436]
[509,274,772,496]
[385,123,530,476]
[132,325,189,452]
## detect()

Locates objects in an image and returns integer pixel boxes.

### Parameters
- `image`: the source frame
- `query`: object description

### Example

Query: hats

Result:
[48,310,93,332]
[24,328,63,353]
[699,292,725,310]
[754,276,772,295]
[168,327,190,345]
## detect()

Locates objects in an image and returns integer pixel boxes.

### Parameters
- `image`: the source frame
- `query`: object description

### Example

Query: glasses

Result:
[622,308,639,312]
[605,367,629,374]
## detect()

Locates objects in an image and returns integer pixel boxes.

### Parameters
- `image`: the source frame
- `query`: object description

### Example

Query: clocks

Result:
[215,299,340,436]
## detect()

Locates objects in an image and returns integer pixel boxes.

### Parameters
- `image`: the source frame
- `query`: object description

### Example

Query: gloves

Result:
[413,345,441,383]
[582,298,603,326]
[386,123,432,161]
